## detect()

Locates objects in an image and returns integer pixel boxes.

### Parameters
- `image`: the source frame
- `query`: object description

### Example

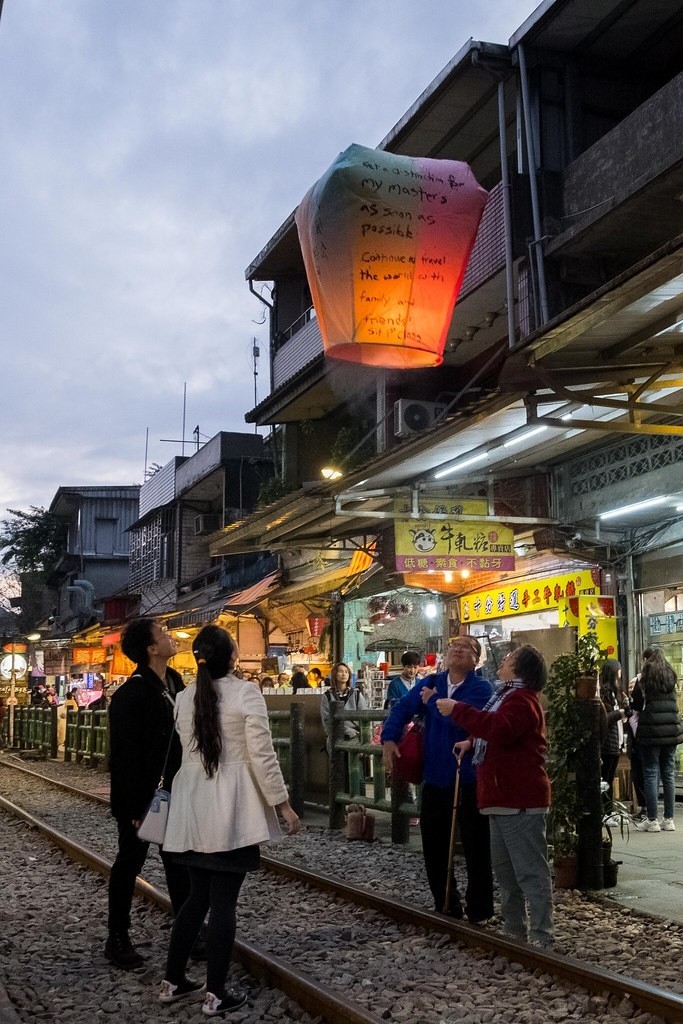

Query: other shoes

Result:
[527,940,545,950]
[505,933,528,941]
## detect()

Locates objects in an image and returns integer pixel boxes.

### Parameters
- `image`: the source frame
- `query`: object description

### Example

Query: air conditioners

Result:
[394,399,448,437]
[193,514,220,534]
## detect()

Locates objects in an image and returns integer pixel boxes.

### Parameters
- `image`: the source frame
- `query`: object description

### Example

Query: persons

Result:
[630,647,683,832]
[202,228,683,556]
[386,652,423,827]
[380,635,496,926]
[157,624,301,1014]
[630,673,648,821]
[106,619,204,969]
[28,684,59,707]
[234,665,312,694]
[600,658,630,826]
[310,668,324,683]
[63,687,79,711]
[437,646,556,948]
[321,662,369,797]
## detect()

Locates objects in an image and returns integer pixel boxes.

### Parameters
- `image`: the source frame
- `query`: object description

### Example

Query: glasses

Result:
[506,650,521,662]
[155,626,168,644]
[448,640,478,656]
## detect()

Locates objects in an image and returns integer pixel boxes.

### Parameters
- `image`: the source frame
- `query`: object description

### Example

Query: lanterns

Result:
[293,144,488,368]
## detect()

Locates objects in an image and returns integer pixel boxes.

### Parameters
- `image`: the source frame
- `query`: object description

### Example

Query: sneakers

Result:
[635,815,661,831]
[159,977,206,1002]
[201,987,248,1015]
[609,812,629,825]
[103,929,143,968]
[190,939,206,961]
[601,814,619,826]
[633,810,649,820]
[658,817,675,830]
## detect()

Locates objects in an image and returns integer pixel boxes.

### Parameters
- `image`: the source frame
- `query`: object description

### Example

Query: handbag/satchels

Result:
[137,791,168,844]
[629,673,646,737]
[392,674,436,785]
[320,738,331,757]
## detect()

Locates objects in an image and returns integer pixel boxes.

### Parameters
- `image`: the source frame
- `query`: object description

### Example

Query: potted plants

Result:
[542,617,624,889]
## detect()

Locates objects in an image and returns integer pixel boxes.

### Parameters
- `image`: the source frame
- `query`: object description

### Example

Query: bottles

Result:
[624,697,629,706]
[665,615,673,633]
[652,617,660,630]
[677,619,683,631]
